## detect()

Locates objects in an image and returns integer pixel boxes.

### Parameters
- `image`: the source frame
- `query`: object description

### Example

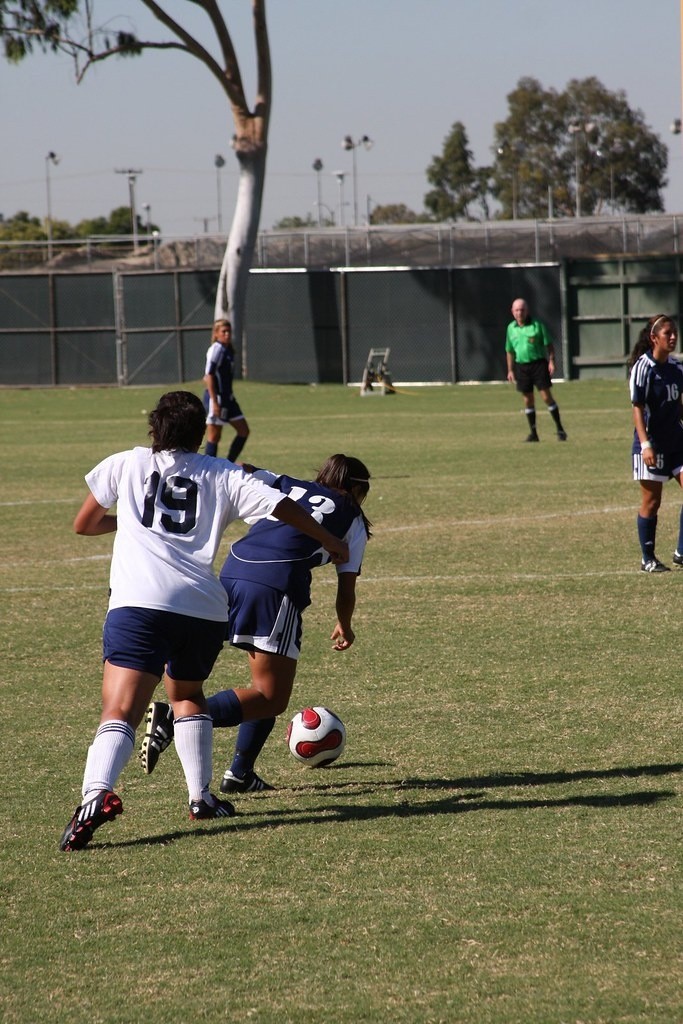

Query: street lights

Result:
[340,133,374,225]
[567,115,596,217]
[331,170,351,227]
[45,151,60,257]
[313,159,322,226]
[141,202,152,244]
[215,154,225,232]
[313,200,349,247]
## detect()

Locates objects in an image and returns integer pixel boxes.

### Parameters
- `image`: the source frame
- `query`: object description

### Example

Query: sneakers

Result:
[60,789,124,852]
[139,701,175,774]
[219,770,276,794]
[189,792,235,819]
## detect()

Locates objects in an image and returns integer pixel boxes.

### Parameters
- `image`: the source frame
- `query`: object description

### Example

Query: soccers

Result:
[286,707,346,768]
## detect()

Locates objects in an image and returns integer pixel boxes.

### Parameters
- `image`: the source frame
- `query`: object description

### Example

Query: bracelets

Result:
[640,441,653,451]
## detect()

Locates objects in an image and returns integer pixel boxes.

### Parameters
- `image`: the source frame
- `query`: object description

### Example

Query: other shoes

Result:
[672,550,683,566]
[640,558,670,573]
[557,431,566,440]
[522,435,539,443]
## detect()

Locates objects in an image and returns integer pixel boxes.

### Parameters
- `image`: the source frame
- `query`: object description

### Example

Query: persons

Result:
[505,297,567,441]
[627,315,683,573]
[202,319,250,464]
[140,453,371,797]
[56,390,350,852]
